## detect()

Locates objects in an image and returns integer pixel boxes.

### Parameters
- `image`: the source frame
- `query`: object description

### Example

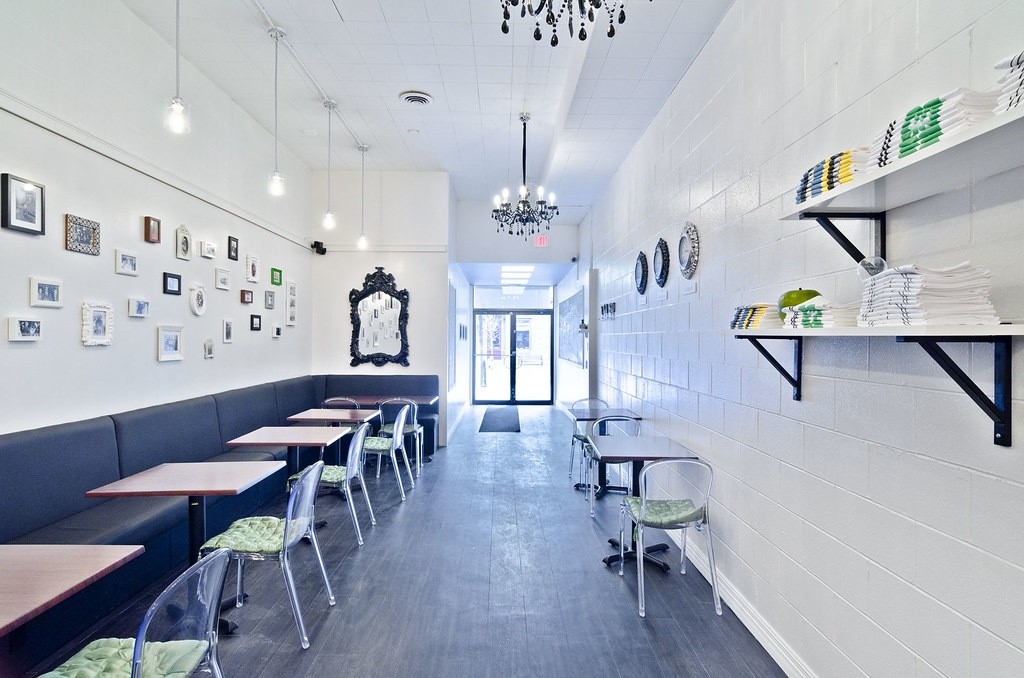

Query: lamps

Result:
[356,143,370,251]
[322,97,338,231]
[166,0,192,135]
[489,113,559,242]
[265,26,287,196]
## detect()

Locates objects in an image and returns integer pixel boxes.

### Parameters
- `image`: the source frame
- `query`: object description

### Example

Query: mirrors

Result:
[348,267,410,367]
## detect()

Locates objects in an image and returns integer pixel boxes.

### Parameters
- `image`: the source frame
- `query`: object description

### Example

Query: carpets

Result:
[478,404,521,432]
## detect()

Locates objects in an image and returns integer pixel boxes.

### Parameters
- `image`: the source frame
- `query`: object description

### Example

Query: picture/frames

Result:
[265,290,275,310]
[114,215,216,296]
[221,319,235,344]
[241,290,253,303]
[246,255,260,282]
[273,326,282,338]
[0,172,101,341]
[215,268,231,291]
[557,286,584,370]
[203,336,216,359]
[286,281,297,327]
[81,298,184,363]
[271,268,283,286]
[251,314,261,330]
[190,283,209,317]
[228,236,239,261]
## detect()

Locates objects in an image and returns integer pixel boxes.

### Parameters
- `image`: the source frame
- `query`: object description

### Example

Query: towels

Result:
[869,88,996,170]
[856,259,1002,326]
[781,295,861,327]
[992,48,1024,116]
[729,303,784,329]
[796,146,870,204]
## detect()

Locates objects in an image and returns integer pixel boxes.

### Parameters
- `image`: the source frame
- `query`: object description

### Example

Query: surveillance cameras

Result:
[314,241,326,255]
[572,258,576,262]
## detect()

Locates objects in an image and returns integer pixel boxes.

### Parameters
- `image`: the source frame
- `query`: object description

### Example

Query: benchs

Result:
[0,374,440,678]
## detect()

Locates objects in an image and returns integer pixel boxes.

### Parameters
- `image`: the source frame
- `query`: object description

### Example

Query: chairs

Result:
[286,422,377,545]
[582,415,641,518]
[377,397,424,479]
[194,460,336,650]
[360,404,415,501]
[37,546,230,678]
[321,396,372,465]
[568,397,609,477]
[618,458,723,618]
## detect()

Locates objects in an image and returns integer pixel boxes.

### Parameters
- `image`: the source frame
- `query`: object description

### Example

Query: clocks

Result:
[678,220,699,281]
[653,238,671,288]
[634,251,649,295]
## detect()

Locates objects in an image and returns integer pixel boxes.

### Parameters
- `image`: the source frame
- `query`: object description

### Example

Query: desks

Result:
[566,408,643,499]
[86,461,286,641]
[287,409,381,501]
[321,395,440,466]
[587,436,699,574]
[225,426,351,528]
[0,544,144,678]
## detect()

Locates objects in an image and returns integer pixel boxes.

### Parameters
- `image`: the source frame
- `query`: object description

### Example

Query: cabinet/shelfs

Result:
[728,103,1024,450]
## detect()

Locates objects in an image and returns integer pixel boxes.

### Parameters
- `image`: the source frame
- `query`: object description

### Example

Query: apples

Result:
[777,288,821,321]
